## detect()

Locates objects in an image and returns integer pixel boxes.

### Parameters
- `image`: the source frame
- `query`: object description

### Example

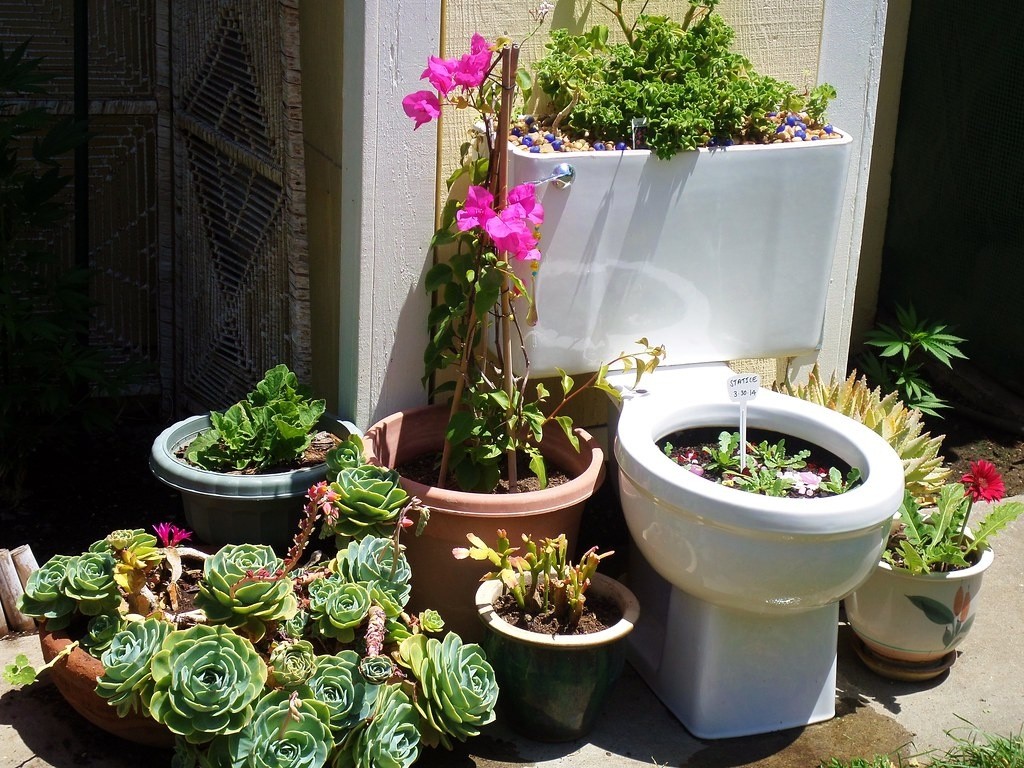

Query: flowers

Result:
[401,0,665,493]
[883,461,1024,575]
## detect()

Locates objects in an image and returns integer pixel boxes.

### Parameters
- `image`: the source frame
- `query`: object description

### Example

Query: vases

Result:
[843,514,994,681]
[363,402,608,646]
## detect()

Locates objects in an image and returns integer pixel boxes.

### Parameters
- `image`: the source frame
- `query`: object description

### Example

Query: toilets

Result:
[473,110,905,739]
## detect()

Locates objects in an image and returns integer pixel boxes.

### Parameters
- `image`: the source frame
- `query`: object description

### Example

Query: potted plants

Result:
[0,0,1024,768]
